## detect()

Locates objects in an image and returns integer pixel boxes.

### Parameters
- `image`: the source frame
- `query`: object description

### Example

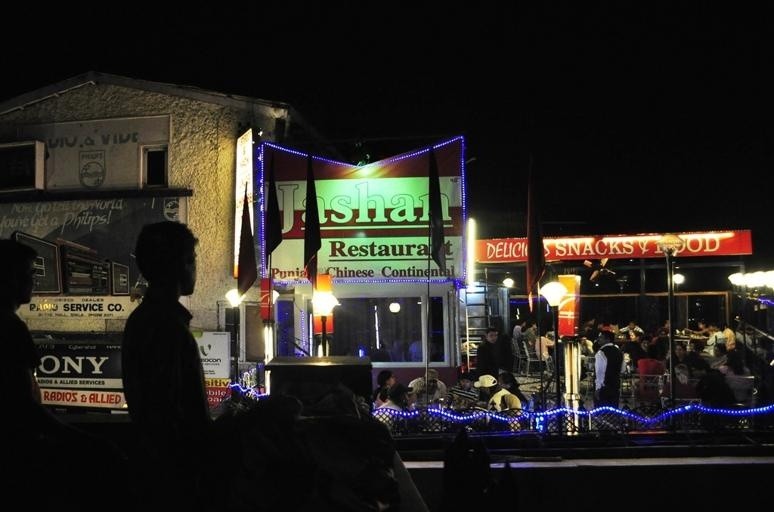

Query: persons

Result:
[227,318,774,512]
[0,239,50,512]
[121,222,212,452]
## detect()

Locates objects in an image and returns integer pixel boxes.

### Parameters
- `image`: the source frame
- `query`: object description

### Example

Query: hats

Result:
[474,375,498,389]
[389,383,413,395]
[424,369,439,381]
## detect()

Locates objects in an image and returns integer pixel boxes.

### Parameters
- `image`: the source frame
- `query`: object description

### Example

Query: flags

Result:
[429,138,447,274]
[304,144,321,287]
[237,192,258,294]
[527,150,547,302]
[265,152,282,269]
